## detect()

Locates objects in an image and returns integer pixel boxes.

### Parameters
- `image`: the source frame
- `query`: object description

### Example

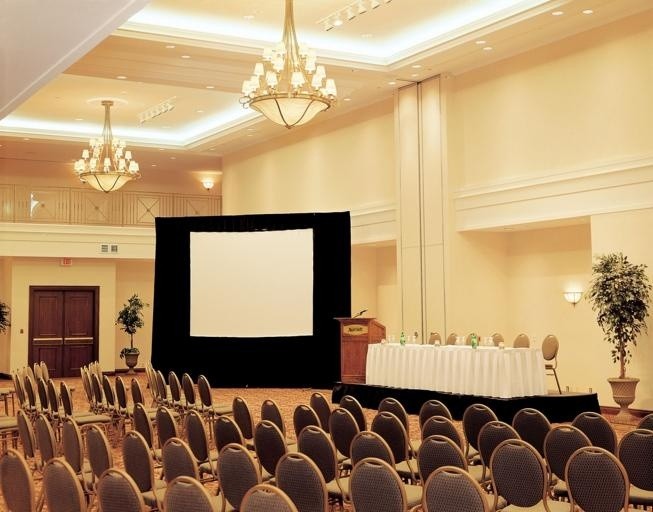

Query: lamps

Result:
[201,179,214,189]
[239,0,338,129]
[74,100,142,193]
[563,291,583,306]
[319,0,391,30]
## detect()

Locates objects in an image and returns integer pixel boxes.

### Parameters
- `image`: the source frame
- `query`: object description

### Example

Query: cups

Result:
[434,340,440,347]
[499,342,505,349]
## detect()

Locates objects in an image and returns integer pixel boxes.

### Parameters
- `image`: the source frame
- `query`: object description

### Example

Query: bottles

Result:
[400,332,405,345]
[472,336,477,349]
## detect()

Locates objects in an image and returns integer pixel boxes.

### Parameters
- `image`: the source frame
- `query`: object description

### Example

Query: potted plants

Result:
[587,253,653,418]
[114,293,149,375]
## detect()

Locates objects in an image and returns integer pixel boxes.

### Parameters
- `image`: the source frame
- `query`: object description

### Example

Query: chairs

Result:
[251,420,287,484]
[59,416,92,492]
[1,334,652,444]
[490,439,577,510]
[162,476,234,510]
[348,456,422,511]
[349,430,422,511]
[133,403,162,468]
[275,453,327,510]
[297,425,350,510]
[41,458,96,511]
[0,449,44,511]
[85,425,114,490]
[34,413,59,477]
[216,443,275,512]
[422,466,507,510]
[546,425,593,503]
[567,446,645,512]
[329,408,360,477]
[14,409,40,472]
[239,484,297,511]
[161,436,226,511]
[616,428,652,512]
[183,410,220,496]
[121,430,168,511]
[369,410,421,484]
[96,467,152,511]
[478,421,521,492]
[417,434,493,492]
